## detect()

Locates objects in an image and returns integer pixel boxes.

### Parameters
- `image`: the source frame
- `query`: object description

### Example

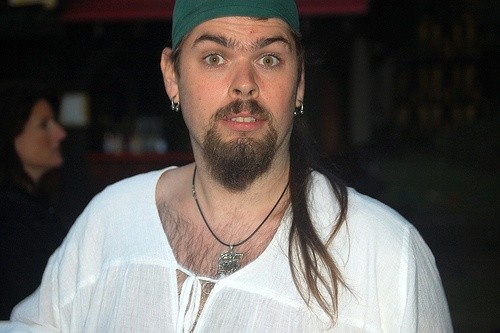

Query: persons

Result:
[0,88,67,322]
[0,0,453,333]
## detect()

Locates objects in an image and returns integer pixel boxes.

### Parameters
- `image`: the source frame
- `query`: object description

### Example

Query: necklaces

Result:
[192,165,289,278]
